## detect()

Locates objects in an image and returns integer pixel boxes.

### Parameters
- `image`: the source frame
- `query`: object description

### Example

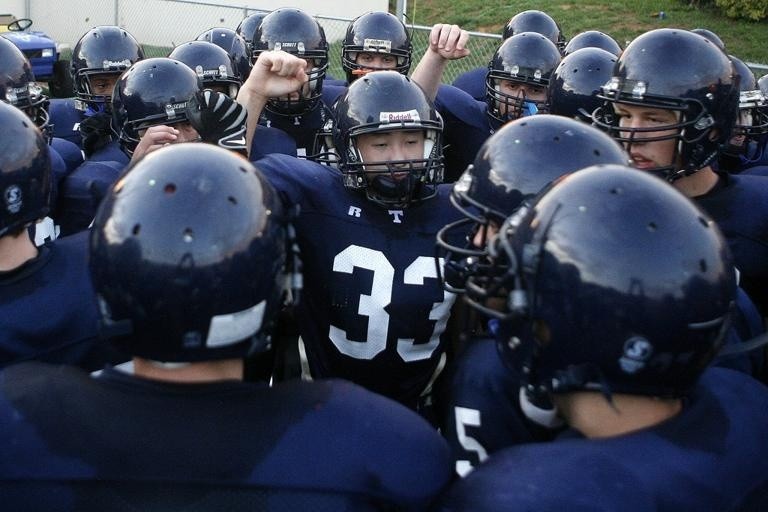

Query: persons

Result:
[0,7,768,511]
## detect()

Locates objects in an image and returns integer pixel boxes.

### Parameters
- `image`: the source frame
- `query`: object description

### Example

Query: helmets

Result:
[90,142,305,361]
[436,113,631,324]
[467,165,737,397]
[338,11,413,87]
[324,70,444,210]
[0,33,52,236]
[69,9,329,155]
